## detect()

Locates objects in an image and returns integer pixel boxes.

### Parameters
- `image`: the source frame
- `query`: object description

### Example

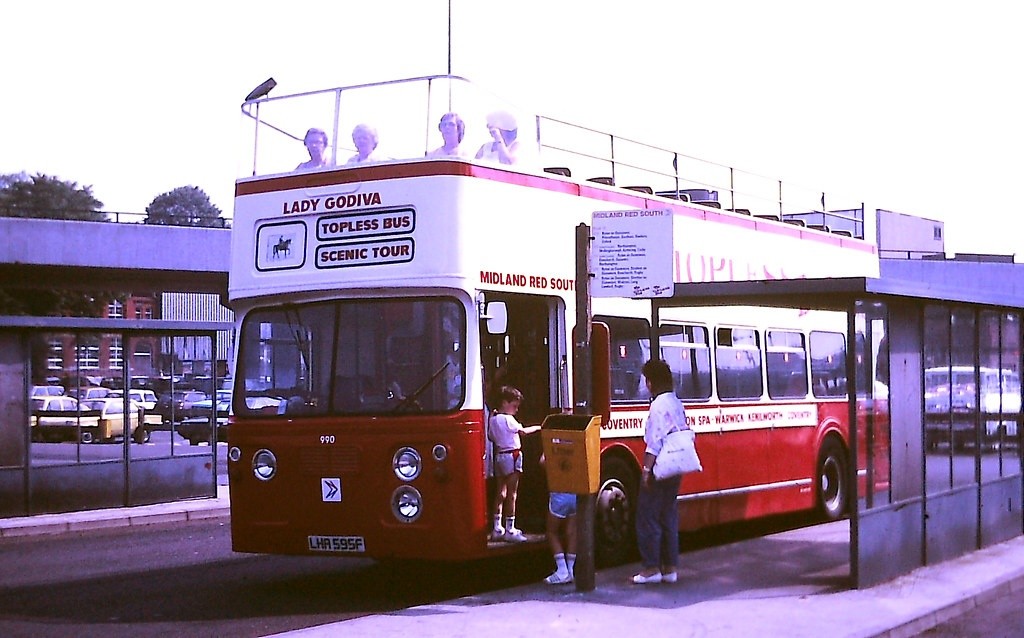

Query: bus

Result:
[219,64,894,575]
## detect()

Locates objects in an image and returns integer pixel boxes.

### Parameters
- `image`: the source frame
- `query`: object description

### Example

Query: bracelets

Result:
[643,465,651,473]
[497,141,504,143]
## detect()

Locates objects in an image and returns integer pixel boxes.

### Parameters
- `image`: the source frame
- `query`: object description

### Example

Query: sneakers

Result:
[661,572,677,583]
[544,572,575,584]
[494,527,527,544]
[632,572,663,584]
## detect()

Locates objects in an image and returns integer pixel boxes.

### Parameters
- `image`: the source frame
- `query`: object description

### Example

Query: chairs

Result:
[545,166,852,240]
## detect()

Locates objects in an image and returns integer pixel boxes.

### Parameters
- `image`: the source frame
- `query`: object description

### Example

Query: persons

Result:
[494,317,552,389]
[294,126,332,169]
[489,386,574,585]
[474,114,518,166]
[629,358,703,583]
[424,112,465,157]
[346,122,384,165]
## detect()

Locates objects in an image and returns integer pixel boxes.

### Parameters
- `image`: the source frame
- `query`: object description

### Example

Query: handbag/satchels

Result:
[647,402,702,482]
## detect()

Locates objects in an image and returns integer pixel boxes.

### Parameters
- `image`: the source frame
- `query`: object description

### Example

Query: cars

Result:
[30,384,231,448]
